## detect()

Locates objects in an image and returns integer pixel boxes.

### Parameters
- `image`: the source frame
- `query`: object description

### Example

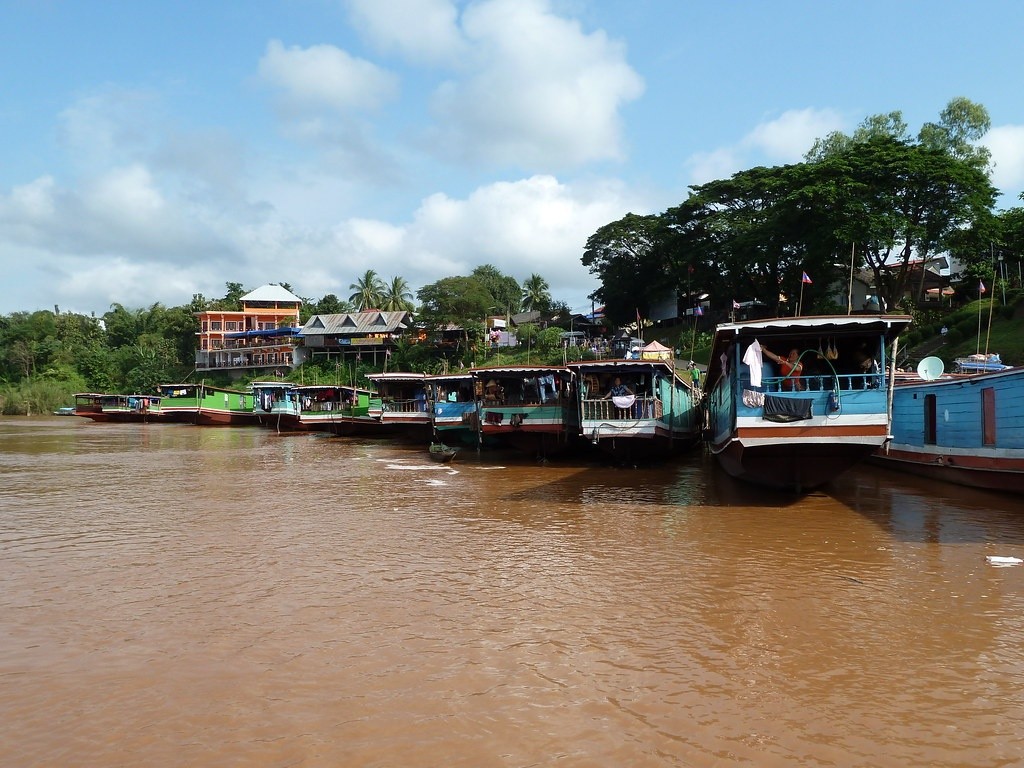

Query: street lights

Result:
[484,305,498,361]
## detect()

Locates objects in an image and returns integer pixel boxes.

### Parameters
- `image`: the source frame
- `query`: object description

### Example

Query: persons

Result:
[688,364,701,389]
[573,337,614,355]
[758,343,804,391]
[484,378,502,404]
[600,377,635,418]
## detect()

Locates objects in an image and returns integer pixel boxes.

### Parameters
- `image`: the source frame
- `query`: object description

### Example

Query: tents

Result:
[227,327,306,345]
[641,340,674,360]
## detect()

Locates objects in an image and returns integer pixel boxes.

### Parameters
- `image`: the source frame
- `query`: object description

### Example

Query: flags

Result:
[637,312,640,320]
[803,271,813,284]
[275,370,285,377]
[693,304,705,316]
[733,300,741,309]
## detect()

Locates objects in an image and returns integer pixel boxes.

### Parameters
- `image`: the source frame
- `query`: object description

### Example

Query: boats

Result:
[865,365,1023,495]
[953,352,1013,373]
[159,383,259,426]
[290,385,381,435]
[466,360,580,454]
[566,359,708,464]
[363,371,439,425]
[103,395,166,419]
[71,392,115,423]
[698,314,915,493]
[420,371,478,440]
[54,407,76,416]
[245,381,319,430]
